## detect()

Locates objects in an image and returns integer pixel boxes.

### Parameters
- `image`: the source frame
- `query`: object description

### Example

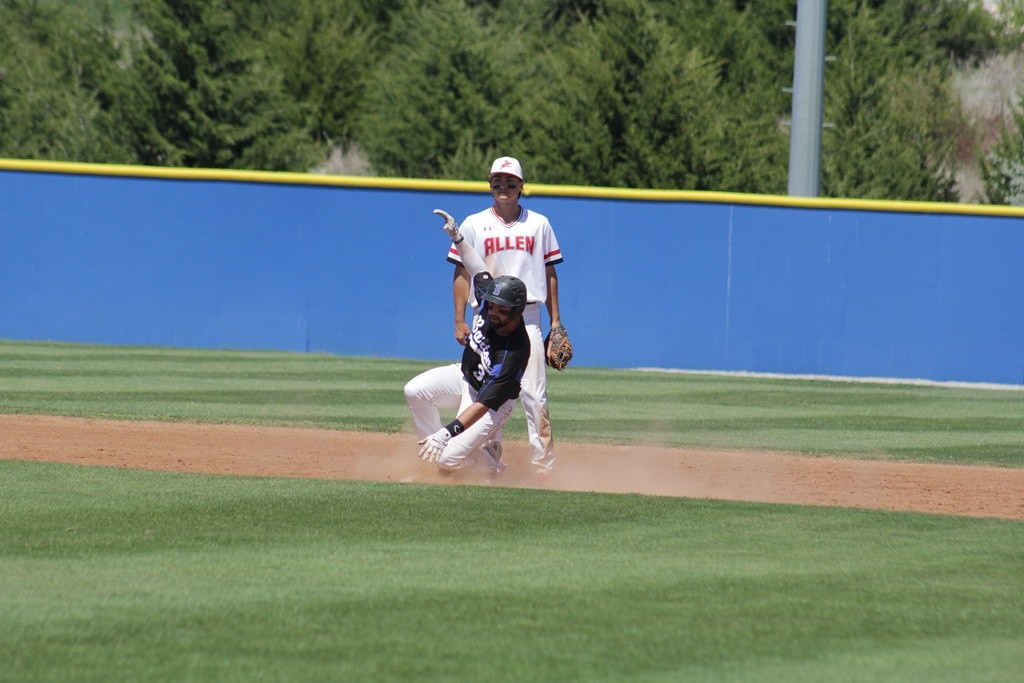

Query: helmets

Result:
[480,275,527,312]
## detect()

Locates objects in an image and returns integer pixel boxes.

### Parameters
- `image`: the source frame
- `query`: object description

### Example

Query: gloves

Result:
[433,210,463,242]
[418,427,451,465]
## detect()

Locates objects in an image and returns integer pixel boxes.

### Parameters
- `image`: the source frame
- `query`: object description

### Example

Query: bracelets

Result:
[445,418,465,438]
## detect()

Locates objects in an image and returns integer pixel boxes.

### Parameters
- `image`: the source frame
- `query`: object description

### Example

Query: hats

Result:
[490,157,522,181]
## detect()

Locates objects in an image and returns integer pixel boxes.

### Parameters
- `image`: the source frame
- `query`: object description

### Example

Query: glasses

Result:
[490,182,519,190]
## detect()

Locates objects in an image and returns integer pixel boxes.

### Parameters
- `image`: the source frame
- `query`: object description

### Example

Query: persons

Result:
[402,155,573,490]
[418,209,531,488]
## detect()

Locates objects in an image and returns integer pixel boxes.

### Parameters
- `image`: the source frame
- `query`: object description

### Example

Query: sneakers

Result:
[484,441,502,476]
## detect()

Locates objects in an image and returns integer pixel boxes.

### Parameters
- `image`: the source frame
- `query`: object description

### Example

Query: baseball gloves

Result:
[542,325,574,372]
